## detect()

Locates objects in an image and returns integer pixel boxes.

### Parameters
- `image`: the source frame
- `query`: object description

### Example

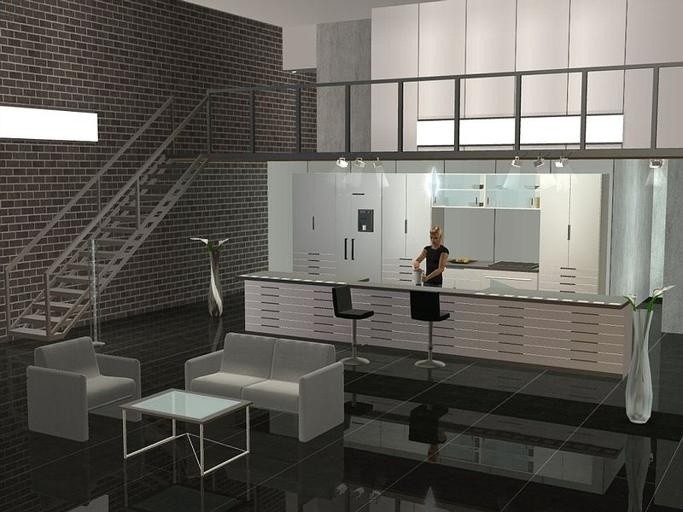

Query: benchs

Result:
[236,270,635,378]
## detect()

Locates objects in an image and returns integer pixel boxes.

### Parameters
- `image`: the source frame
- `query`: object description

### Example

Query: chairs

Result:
[332,284,375,364]
[409,288,451,368]
[26,336,142,442]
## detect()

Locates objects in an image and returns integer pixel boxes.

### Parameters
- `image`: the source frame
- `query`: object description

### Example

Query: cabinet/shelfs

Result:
[538,174,609,296]
[442,268,538,294]
[291,173,336,278]
[433,173,539,211]
[381,173,443,287]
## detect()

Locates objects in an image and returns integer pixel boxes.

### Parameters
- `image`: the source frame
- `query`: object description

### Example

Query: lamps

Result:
[555,158,568,168]
[648,158,663,169]
[534,156,545,168]
[512,156,521,167]
[336,155,383,169]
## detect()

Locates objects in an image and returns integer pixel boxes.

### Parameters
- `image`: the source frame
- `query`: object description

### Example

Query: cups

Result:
[422,274,427,282]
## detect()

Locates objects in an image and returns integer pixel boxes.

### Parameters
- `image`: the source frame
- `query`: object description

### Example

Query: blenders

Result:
[414,269,422,287]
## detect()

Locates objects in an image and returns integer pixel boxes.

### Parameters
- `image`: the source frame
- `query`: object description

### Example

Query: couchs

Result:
[184,330,344,442]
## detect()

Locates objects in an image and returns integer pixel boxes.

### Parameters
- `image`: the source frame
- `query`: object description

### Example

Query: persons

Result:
[412,226,449,287]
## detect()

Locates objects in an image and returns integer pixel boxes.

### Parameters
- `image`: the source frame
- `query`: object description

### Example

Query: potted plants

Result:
[189,233,231,318]
[623,281,677,425]
